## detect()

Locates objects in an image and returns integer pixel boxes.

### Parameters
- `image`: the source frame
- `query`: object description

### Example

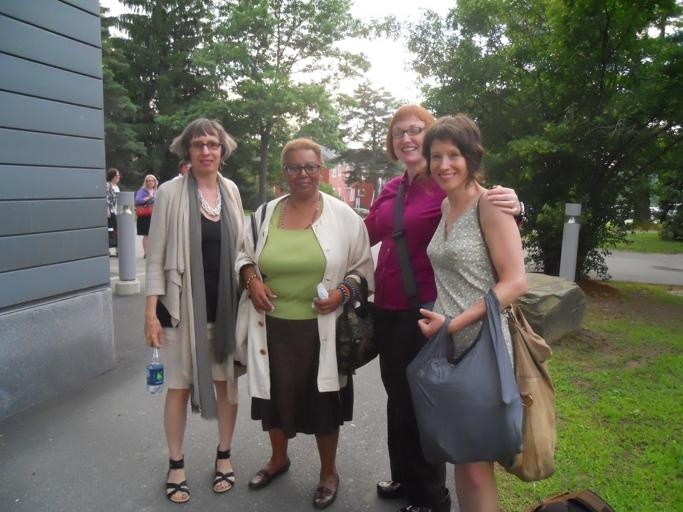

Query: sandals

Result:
[213,444,235,492]
[167,454,191,503]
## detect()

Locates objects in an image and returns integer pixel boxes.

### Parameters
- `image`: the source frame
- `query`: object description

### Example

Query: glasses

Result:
[392,127,425,138]
[284,165,321,174]
[188,142,223,150]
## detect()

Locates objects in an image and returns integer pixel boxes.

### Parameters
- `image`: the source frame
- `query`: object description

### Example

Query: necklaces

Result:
[279,190,322,232]
[194,182,224,219]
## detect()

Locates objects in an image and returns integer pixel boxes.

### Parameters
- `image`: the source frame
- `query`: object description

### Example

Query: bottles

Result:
[144,344,165,396]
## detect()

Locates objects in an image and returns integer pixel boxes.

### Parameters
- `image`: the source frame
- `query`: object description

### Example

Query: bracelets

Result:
[340,279,356,302]
[335,285,348,306]
[245,273,261,291]
[515,199,526,227]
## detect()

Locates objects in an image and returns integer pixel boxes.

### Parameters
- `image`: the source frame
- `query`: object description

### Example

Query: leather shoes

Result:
[248,458,290,489]
[377,481,403,498]
[313,473,339,507]
[399,488,451,512]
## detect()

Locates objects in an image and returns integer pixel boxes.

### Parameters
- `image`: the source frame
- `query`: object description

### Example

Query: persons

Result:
[143,117,245,504]
[415,114,527,512]
[133,173,161,262]
[106,167,122,246]
[358,101,527,511]
[234,138,376,508]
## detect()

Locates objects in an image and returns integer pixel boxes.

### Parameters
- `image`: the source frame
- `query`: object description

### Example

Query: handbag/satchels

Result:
[506,306,556,482]
[136,189,153,217]
[336,278,376,375]
[108,218,117,247]
[405,289,523,468]
